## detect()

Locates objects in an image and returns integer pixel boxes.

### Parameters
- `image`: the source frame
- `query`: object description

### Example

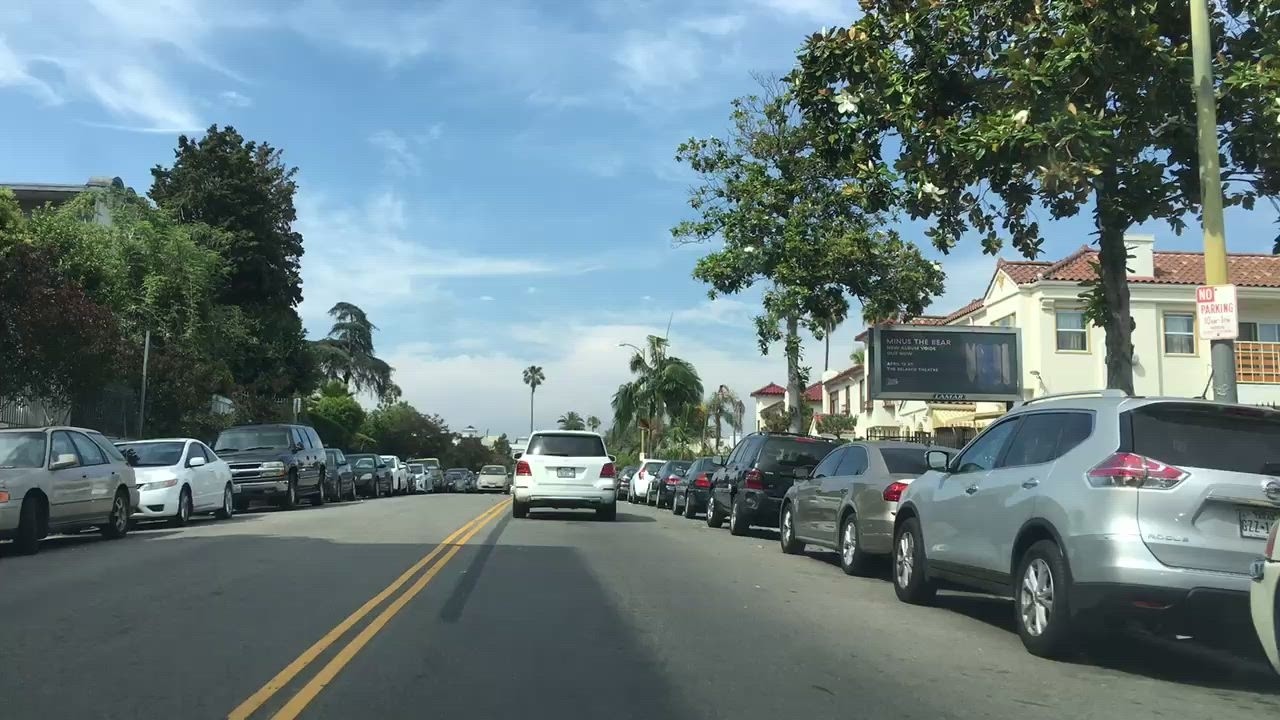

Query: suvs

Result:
[704,431,846,538]
[626,459,669,505]
[616,466,639,500]
[891,390,1280,661]
[672,456,728,520]
[209,423,327,511]
[511,429,617,522]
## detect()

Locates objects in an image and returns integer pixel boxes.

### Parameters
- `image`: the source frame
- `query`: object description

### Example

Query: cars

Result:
[324,448,357,503]
[344,453,394,499]
[778,440,963,575]
[353,455,408,496]
[405,457,478,493]
[408,463,434,494]
[1249,520,1280,676]
[112,437,234,528]
[0,425,140,555]
[401,462,417,495]
[647,459,694,508]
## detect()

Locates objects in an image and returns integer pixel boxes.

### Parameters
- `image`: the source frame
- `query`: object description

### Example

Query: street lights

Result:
[616,342,647,408]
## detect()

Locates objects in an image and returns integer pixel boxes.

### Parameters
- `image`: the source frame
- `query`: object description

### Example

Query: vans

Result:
[475,465,513,495]
